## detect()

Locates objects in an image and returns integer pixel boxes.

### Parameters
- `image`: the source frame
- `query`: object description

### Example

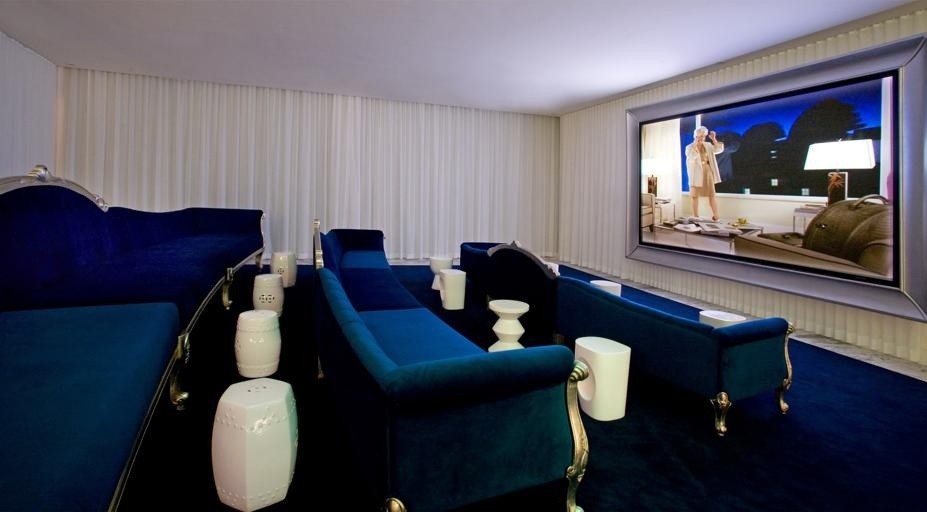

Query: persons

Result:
[685,126,724,222]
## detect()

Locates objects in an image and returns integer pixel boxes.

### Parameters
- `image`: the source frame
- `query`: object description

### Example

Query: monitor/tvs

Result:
[638,68,902,290]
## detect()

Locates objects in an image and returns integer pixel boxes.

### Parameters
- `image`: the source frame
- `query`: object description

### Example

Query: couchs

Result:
[2,163,265,512]
[310,218,592,512]
[459,239,795,436]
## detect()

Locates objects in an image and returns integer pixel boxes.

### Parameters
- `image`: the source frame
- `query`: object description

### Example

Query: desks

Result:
[486,298,531,354]
[428,255,454,291]
[573,335,632,423]
[591,279,621,298]
[438,268,466,310]
[546,262,560,276]
[698,310,748,329]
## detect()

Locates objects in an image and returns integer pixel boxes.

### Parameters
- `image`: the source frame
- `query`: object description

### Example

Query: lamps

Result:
[801,137,877,198]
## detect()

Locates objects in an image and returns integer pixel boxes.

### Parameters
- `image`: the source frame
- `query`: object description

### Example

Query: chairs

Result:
[640,193,656,234]
[735,194,894,280]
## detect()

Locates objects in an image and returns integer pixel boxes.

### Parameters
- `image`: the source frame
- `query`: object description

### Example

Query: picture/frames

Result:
[623,31,927,325]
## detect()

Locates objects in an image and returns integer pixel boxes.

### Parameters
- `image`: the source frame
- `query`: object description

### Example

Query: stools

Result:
[210,250,298,512]
